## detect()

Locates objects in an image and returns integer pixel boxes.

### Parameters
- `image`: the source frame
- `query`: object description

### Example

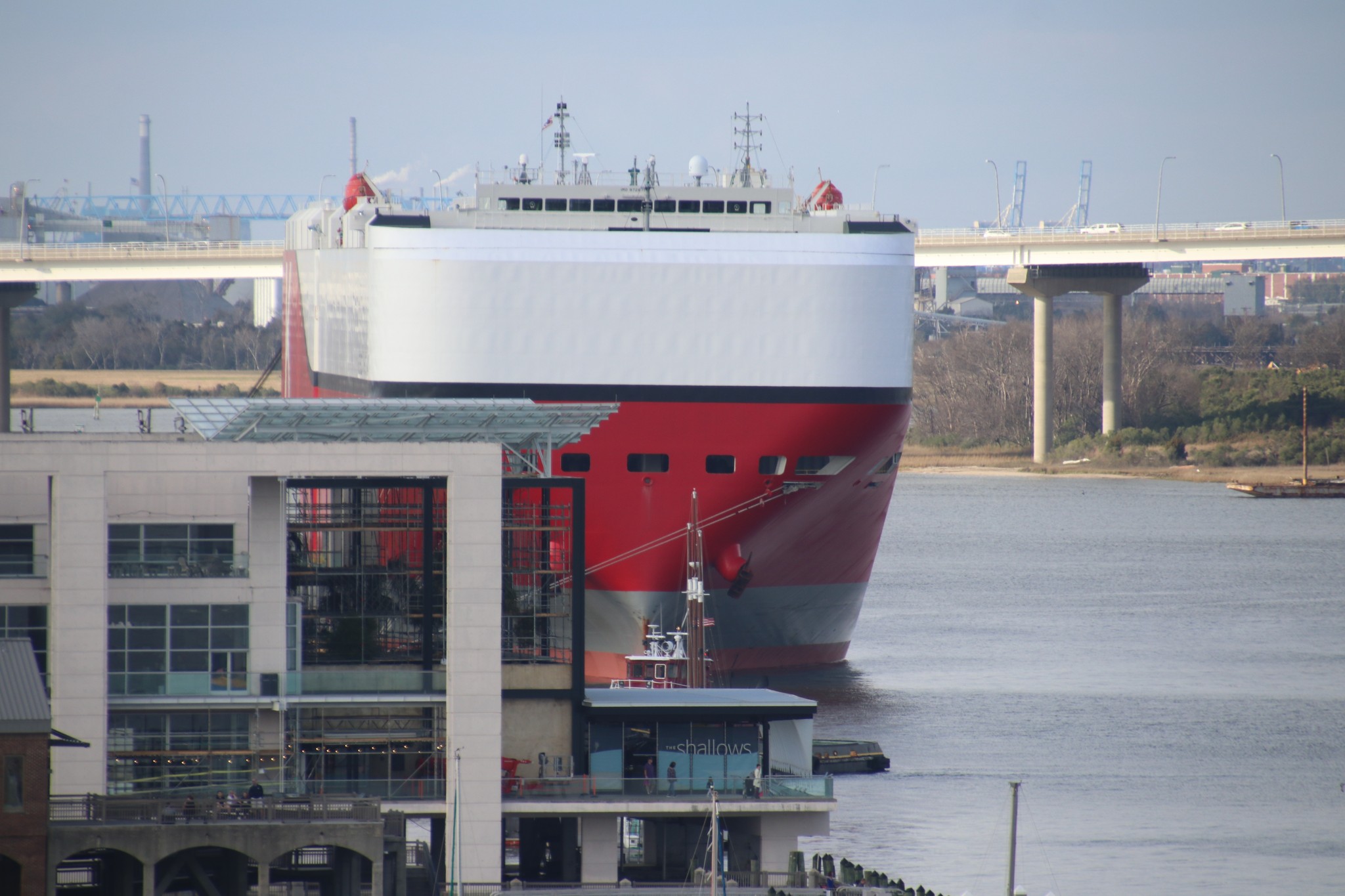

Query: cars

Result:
[1214,222,1251,231]
[1290,220,1319,230]
[983,229,1011,238]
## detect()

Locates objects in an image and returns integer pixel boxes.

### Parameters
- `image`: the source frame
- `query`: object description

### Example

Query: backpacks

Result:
[749,769,759,780]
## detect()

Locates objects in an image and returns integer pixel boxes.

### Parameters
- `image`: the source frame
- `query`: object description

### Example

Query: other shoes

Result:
[667,793,676,797]
[647,792,653,797]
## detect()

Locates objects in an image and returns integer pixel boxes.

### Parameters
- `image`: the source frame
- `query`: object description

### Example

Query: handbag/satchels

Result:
[758,786,762,794]
[710,785,714,788]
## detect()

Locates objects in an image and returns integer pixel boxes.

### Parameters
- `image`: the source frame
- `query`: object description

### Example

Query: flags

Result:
[542,115,553,129]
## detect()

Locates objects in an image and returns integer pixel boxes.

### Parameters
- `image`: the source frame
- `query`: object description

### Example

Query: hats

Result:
[648,757,654,760]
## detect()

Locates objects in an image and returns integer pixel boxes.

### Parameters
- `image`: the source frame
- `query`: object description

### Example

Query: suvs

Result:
[1080,223,1122,235]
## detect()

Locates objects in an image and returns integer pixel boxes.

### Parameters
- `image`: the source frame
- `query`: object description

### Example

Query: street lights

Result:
[1270,154,1286,222]
[1155,156,1176,239]
[985,159,1001,229]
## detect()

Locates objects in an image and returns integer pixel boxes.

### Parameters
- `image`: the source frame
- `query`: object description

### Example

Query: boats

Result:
[0,149,918,691]
